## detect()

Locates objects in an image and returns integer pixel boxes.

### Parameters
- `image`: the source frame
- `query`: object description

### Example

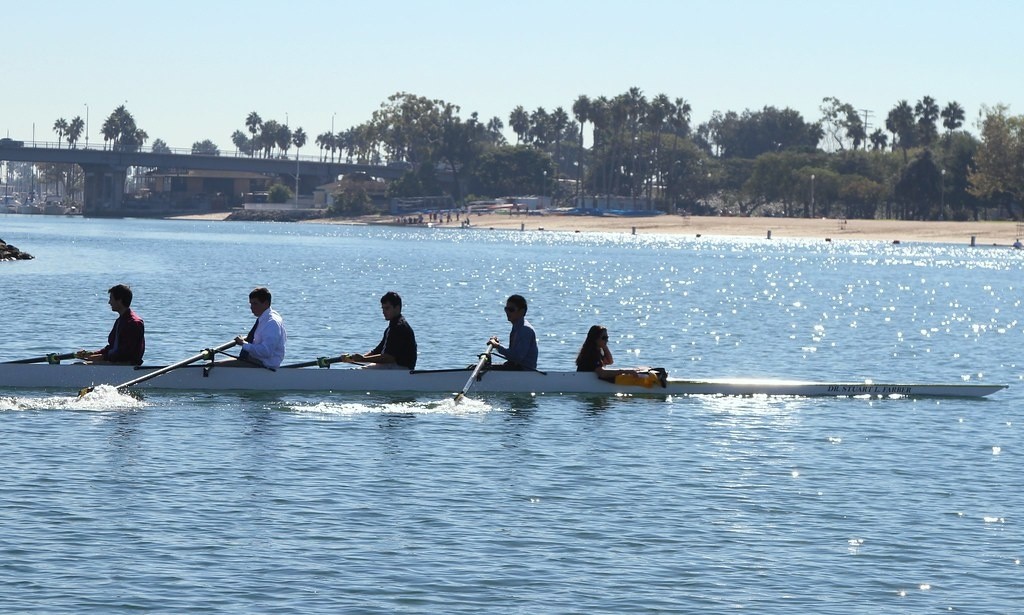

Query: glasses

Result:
[504,306,521,312]
[599,335,609,340]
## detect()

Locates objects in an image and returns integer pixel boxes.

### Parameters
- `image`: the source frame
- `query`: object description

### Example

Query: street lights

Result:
[542,170,547,208]
[940,169,946,219]
[811,174,816,219]
[83,102,89,148]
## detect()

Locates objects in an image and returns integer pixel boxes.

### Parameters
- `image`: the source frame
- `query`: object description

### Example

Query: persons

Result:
[469,293,539,370]
[348,291,417,370]
[206,288,287,370]
[391,202,533,230]
[575,324,614,373]
[1014,238,1024,249]
[72,284,147,366]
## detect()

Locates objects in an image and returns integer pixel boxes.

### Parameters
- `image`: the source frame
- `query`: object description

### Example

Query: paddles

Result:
[278,351,359,369]
[77,334,248,402]
[449,334,498,408]
[0,348,94,364]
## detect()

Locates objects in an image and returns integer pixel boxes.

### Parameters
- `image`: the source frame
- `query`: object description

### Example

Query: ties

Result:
[113,322,120,353]
[240,318,259,360]
[381,327,390,356]
[510,328,515,351]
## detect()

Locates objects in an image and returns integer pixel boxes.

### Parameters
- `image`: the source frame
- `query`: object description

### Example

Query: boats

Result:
[0,352,1010,399]
